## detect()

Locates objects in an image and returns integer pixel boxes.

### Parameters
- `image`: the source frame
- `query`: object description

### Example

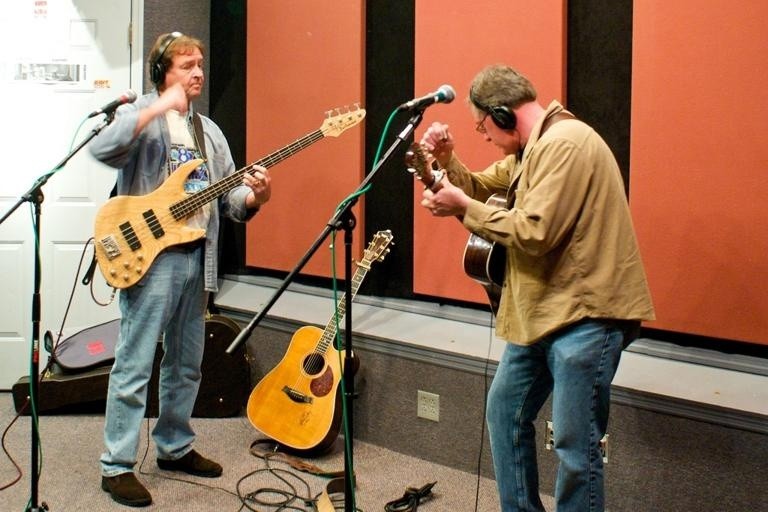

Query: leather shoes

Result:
[101,472,151,507]
[156,449,222,478]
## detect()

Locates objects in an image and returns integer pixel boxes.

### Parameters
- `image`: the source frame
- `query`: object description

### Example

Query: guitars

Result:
[247,229,395,459]
[404,142,508,318]
[95,102,367,289]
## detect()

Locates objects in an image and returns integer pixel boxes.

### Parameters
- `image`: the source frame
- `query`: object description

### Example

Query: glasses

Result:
[472,113,487,134]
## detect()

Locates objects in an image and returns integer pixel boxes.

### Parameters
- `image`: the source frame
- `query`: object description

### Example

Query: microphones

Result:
[401,84,457,116]
[89,88,138,121]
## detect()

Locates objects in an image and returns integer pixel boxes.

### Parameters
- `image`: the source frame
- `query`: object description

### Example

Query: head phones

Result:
[149,31,180,90]
[468,82,517,134]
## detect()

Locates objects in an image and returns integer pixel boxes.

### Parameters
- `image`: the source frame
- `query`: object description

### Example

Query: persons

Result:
[416,65,656,512]
[90,30,272,507]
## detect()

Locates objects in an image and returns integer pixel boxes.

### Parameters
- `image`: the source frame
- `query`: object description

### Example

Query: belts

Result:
[165,240,200,254]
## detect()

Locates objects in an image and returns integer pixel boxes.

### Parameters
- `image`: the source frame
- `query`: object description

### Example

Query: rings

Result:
[254,181,261,187]
